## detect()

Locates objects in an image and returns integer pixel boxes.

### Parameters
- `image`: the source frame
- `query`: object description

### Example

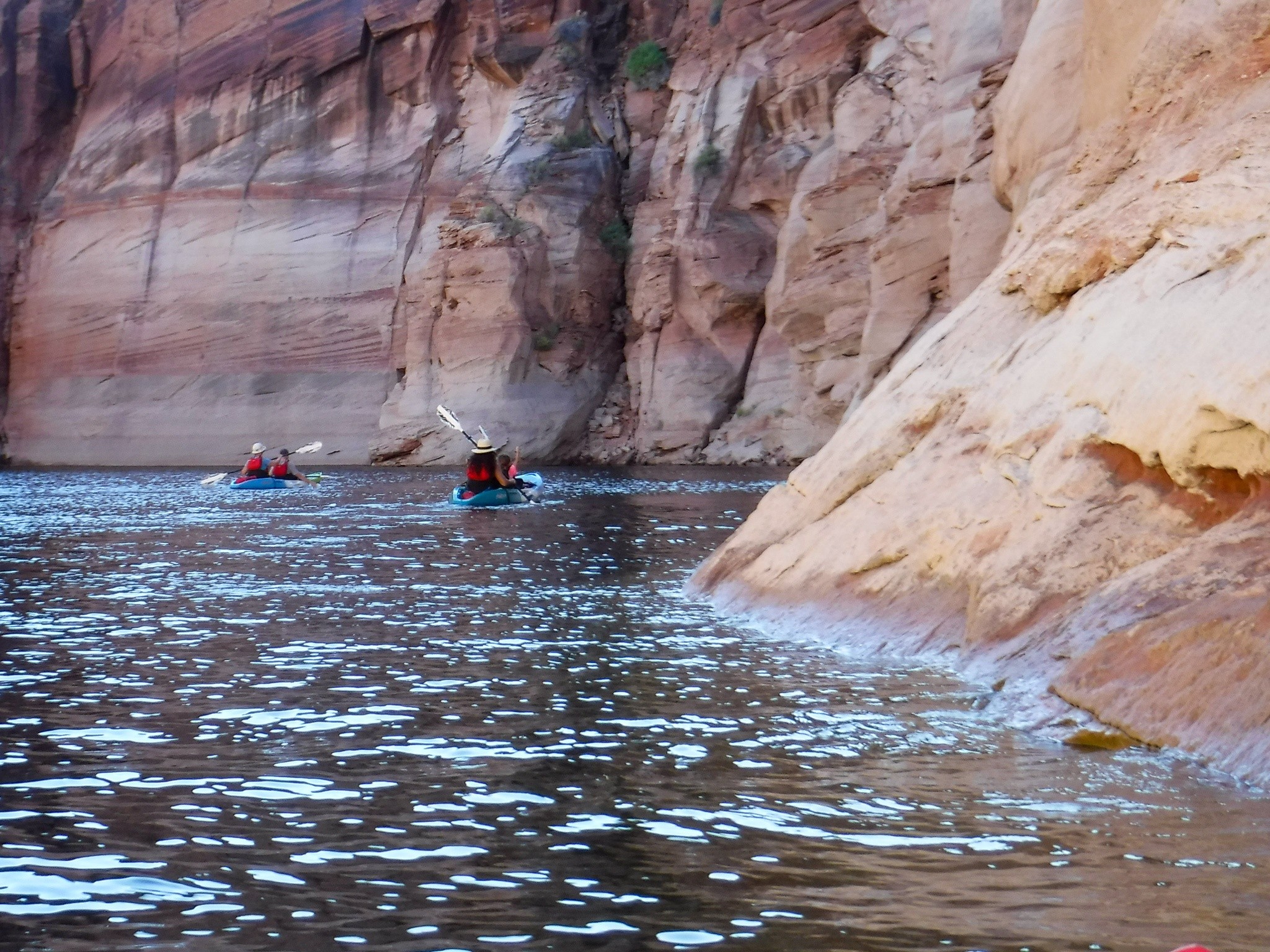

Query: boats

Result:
[230,473,323,488]
[447,471,545,505]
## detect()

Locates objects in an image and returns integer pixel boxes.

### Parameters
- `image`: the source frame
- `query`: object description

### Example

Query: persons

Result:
[241,443,316,487]
[465,439,533,495]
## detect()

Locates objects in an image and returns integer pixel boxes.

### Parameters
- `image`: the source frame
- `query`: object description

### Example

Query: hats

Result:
[471,438,498,453]
[280,449,289,455]
[251,443,267,454]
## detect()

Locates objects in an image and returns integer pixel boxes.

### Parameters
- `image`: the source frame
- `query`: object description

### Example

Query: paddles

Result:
[304,475,345,478]
[436,404,540,504]
[200,441,322,487]
